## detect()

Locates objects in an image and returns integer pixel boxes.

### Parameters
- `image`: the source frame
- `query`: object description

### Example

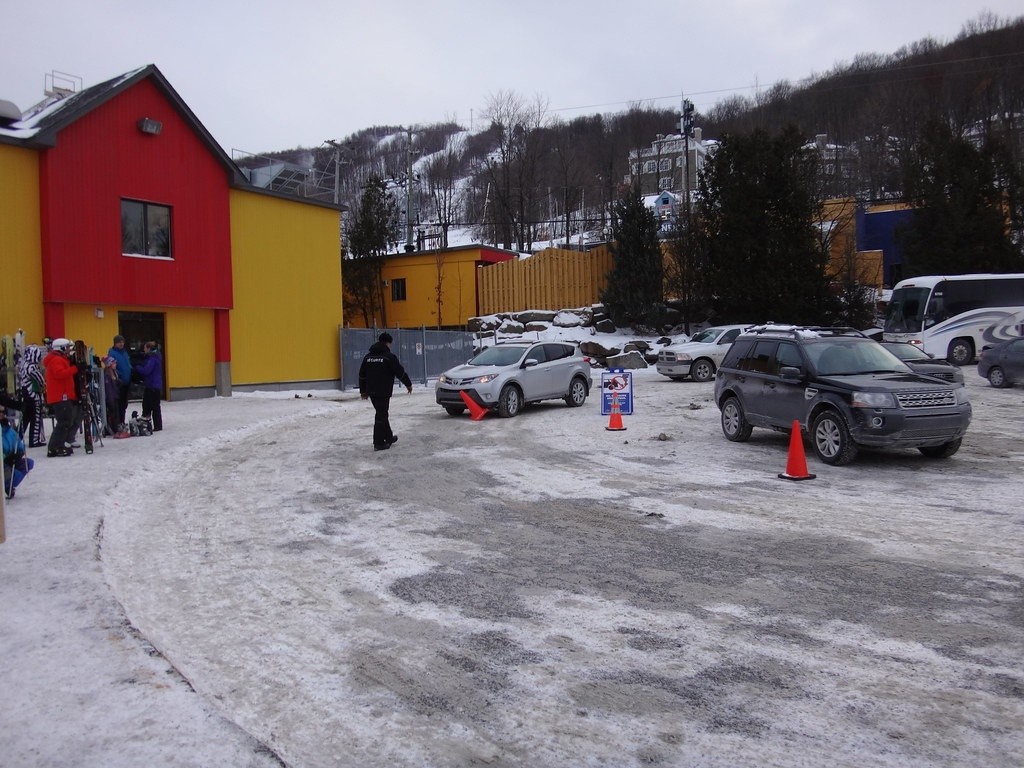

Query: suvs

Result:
[436,341,592,418]
[714,326,973,466]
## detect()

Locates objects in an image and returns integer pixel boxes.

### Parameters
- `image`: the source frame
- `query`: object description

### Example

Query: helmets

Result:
[52,338,75,353]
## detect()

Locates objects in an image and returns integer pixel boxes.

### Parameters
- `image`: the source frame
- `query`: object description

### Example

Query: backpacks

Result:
[129,412,152,436]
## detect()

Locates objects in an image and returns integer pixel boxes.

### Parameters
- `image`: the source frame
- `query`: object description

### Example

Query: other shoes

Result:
[5,487,15,497]
[64,442,80,448]
[29,441,46,448]
[47,448,73,457]
[374,436,398,451]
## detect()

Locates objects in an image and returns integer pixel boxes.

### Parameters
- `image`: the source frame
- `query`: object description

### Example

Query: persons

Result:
[42,338,93,457]
[358,333,413,451]
[0,406,34,499]
[0,328,59,402]
[135,341,162,431]
[17,344,48,447]
[101,335,133,434]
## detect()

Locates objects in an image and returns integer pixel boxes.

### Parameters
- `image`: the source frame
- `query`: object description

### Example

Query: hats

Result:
[102,356,117,367]
[113,335,125,342]
[379,333,393,343]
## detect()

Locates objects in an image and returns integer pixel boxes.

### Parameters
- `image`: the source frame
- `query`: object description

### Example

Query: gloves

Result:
[76,362,86,371]
[4,453,21,468]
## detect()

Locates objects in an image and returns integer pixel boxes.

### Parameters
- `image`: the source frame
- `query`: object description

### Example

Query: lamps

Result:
[137,117,163,136]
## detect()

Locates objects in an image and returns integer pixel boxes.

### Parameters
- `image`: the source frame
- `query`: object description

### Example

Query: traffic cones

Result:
[778,420,817,481]
[604,392,628,431]
[460,391,489,421]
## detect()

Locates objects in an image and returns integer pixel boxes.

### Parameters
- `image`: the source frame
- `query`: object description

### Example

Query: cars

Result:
[656,324,755,382]
[977,336,1024,389]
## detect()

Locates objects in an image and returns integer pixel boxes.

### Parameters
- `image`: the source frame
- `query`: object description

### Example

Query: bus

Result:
[882,273,1024,365]
